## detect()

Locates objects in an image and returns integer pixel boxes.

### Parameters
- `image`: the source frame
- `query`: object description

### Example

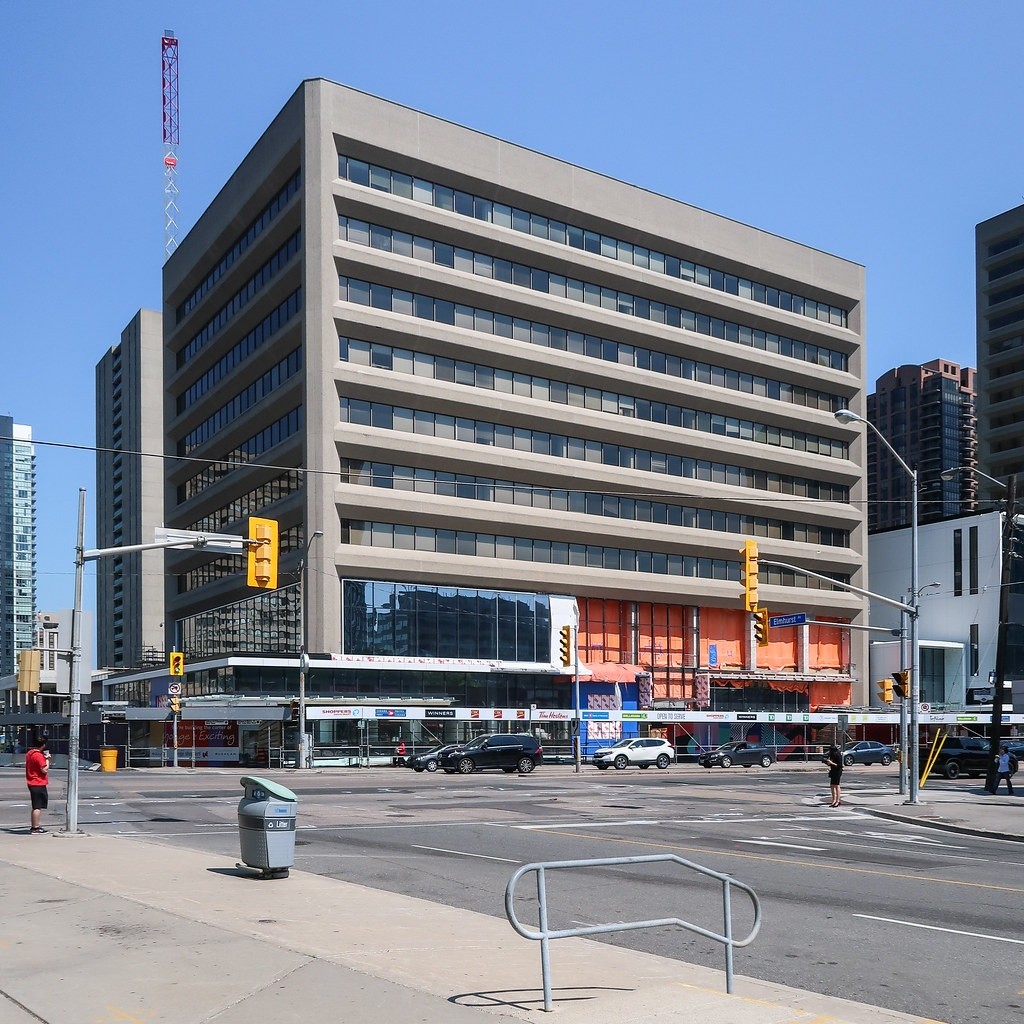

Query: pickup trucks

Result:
[909,736,1018,780]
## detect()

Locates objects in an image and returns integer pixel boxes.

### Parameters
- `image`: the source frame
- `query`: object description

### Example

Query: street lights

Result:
[835,409,925,807]
[940,467,1017,791]
[300,531,326,768]
[894,583,940,796]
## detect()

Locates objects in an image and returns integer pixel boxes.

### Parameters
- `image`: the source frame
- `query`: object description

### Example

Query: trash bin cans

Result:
[99,746,118,771]
[236,776,297,877]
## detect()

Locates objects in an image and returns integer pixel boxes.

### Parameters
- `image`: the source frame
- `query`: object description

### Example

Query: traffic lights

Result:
[170,653,184,676]
[753,607,769,647]
[876,679,892,704]
[169,697,179,712]
[891,671,911,699]
[559,626,570,666]
[738,539,759,613]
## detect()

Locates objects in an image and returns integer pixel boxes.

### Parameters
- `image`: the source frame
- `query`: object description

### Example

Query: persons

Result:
[822,745,843,807]
[15,742,23,754]
[990,746,1015,794]
[396,738,407,768]
[5,742,14,754]
[25,736,52,834]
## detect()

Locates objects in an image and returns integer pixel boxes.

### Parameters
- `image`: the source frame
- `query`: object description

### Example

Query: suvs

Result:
[437,734,543,774]
[592,738,674,770]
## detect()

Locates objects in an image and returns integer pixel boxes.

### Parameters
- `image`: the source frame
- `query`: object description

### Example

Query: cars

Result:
[698,741,776,768]
[984,740,1024,759]
[405,744,465,773]
[823,741,895,766]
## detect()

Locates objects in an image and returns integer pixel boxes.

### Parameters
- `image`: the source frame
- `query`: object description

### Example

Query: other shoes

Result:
[30,828,48,834]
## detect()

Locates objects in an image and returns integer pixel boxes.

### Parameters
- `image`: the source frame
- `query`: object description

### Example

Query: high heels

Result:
[829,802,836,807]
[834,802,841,807]
